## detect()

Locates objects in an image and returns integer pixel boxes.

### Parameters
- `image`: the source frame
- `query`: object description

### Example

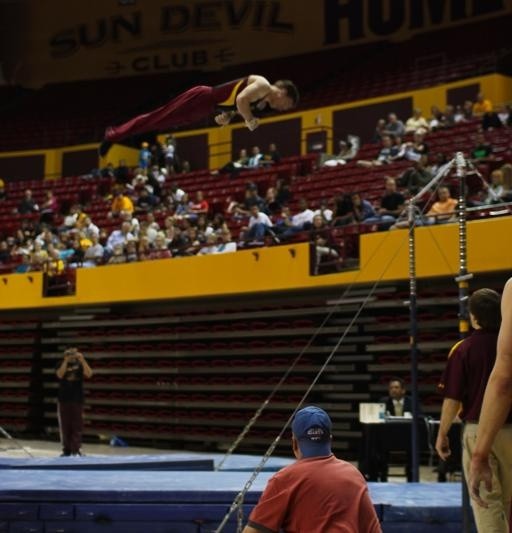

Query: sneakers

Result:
[59,451,81,457]
[100,138,113,156]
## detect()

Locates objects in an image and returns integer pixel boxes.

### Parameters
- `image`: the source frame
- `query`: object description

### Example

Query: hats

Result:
[292,406,333,456]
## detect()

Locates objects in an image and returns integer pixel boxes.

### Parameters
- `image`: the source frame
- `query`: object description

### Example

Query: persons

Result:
[53,344,93,458]
[243,405,384,532]
[468,273,512,507]
[97,74,299,157]
[431,282,512,533]
[380,380,430,484]
[0,99,510,277]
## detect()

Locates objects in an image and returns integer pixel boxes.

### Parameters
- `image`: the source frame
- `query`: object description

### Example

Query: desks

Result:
[353,416,461,482]
